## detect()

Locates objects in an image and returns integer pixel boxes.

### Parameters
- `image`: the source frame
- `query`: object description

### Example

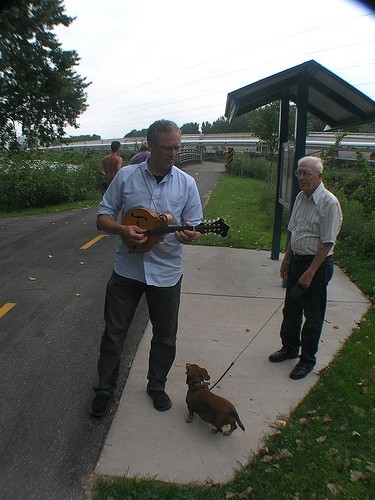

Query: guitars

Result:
[121,208,230,254]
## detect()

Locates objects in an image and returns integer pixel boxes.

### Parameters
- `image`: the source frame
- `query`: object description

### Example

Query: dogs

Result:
[184,362,246,436]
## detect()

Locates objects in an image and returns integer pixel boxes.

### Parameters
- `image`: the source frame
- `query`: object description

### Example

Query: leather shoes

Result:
[269,350,298,362]
[290,361,313,380]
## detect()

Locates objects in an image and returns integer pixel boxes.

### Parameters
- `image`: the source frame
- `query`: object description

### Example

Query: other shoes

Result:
[90,394,109,416]
[146,387,172,411]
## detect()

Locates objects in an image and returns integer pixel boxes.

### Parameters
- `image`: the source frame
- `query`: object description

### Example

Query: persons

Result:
[99,141,122,196]
[130,141,151,165]
[269,156,343,380]
[89,120,203,416]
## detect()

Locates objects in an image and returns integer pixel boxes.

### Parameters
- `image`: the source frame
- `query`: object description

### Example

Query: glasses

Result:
[150,140,181,152]
[294,169,319,177]
